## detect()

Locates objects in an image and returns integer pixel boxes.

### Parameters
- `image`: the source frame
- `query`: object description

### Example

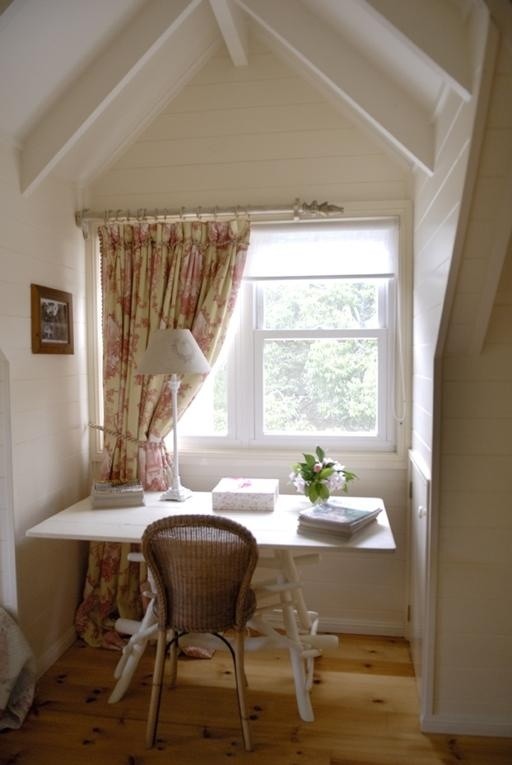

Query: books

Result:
[90,481,145,509]
[296,502,383,544]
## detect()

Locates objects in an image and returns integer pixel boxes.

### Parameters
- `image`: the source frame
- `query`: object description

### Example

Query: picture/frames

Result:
[25,279,76,357]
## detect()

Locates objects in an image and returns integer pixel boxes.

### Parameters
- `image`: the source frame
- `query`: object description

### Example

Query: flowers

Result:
[287,443,359,505]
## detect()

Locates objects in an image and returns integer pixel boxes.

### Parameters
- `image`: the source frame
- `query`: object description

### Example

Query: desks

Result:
[23,481,404,725]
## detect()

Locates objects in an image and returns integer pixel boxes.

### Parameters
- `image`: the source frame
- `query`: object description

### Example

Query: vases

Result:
[310,496,325,506]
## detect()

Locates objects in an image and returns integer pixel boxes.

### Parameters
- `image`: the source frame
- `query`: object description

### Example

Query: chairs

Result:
[139,514,263,753]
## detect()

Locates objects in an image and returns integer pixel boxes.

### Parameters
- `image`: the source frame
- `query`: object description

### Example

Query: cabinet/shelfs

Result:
[406,448,427,732]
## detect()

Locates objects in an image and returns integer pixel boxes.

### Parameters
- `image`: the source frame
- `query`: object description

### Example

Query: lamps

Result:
[130,325,217,507]
